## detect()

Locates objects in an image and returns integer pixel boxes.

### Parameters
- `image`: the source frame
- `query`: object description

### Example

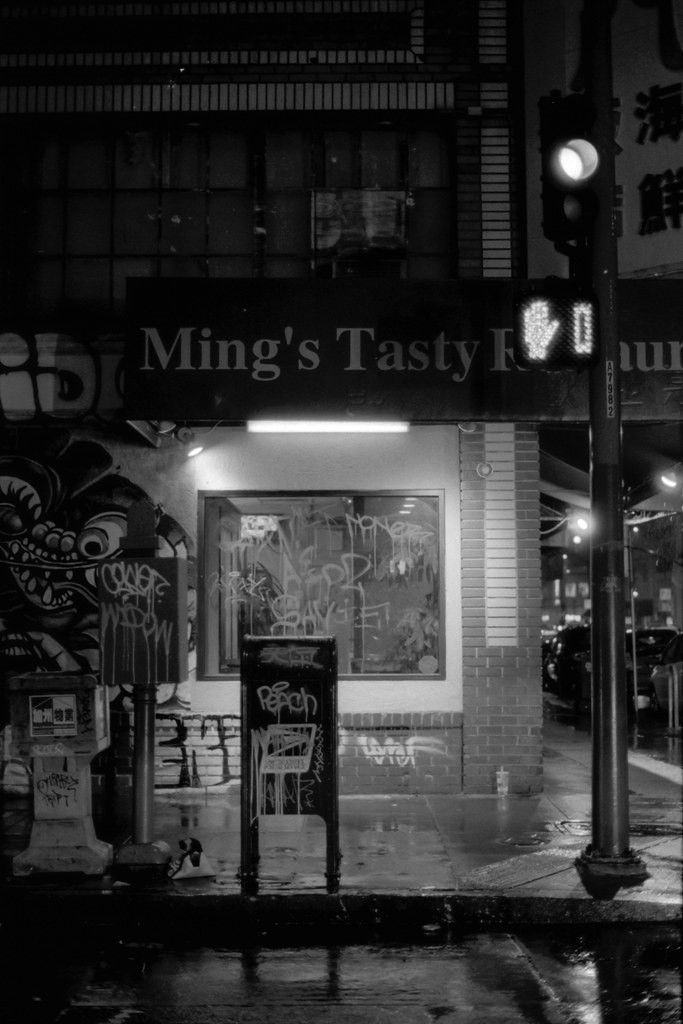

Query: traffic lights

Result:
[538,88,612,243]
[519,275,604,370]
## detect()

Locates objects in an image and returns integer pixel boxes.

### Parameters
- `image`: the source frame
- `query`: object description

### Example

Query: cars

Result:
[544,623,682,731]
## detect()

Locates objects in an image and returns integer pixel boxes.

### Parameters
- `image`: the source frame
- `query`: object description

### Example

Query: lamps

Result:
[183,436,204,458]
[158,422,176,433]
